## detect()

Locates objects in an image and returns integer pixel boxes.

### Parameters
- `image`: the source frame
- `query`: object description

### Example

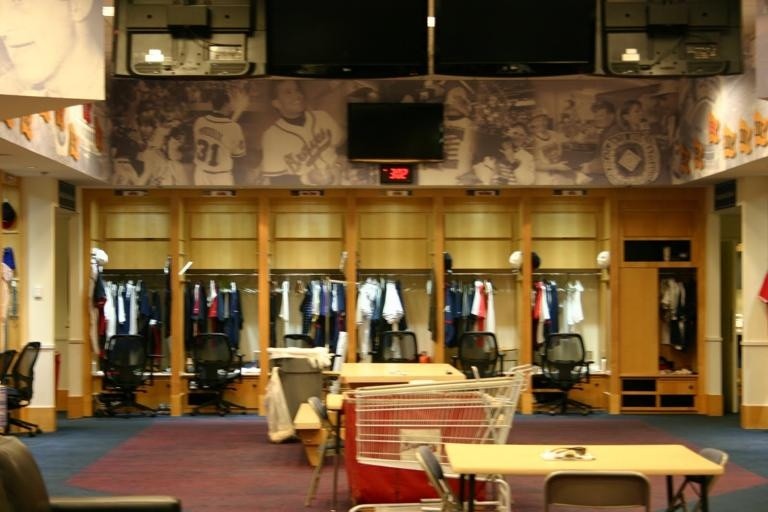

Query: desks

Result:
[443,441,724,512]
[328,359,467,501]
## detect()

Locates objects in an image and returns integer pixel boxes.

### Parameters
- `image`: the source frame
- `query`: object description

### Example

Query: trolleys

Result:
[342,364,538,512]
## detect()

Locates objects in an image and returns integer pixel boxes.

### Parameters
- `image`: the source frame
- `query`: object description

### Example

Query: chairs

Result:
[542,470,654,512]
[414,446,512,511]
[1,341,44,436]
[665,447,730,511]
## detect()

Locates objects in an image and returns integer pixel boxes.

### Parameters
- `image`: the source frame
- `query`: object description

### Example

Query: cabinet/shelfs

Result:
[85,184,712,422]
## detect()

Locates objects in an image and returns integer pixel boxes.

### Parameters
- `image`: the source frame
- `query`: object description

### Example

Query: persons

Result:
[0,0,105,100]
[400,81,709,185]
[110,81,373,187]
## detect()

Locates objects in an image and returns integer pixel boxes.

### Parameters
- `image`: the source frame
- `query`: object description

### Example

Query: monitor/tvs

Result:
[434,0,595,77]
[269,1,429,81]
[594,0,743,78]
[346,102,445,165]
[109,0,268,79]
